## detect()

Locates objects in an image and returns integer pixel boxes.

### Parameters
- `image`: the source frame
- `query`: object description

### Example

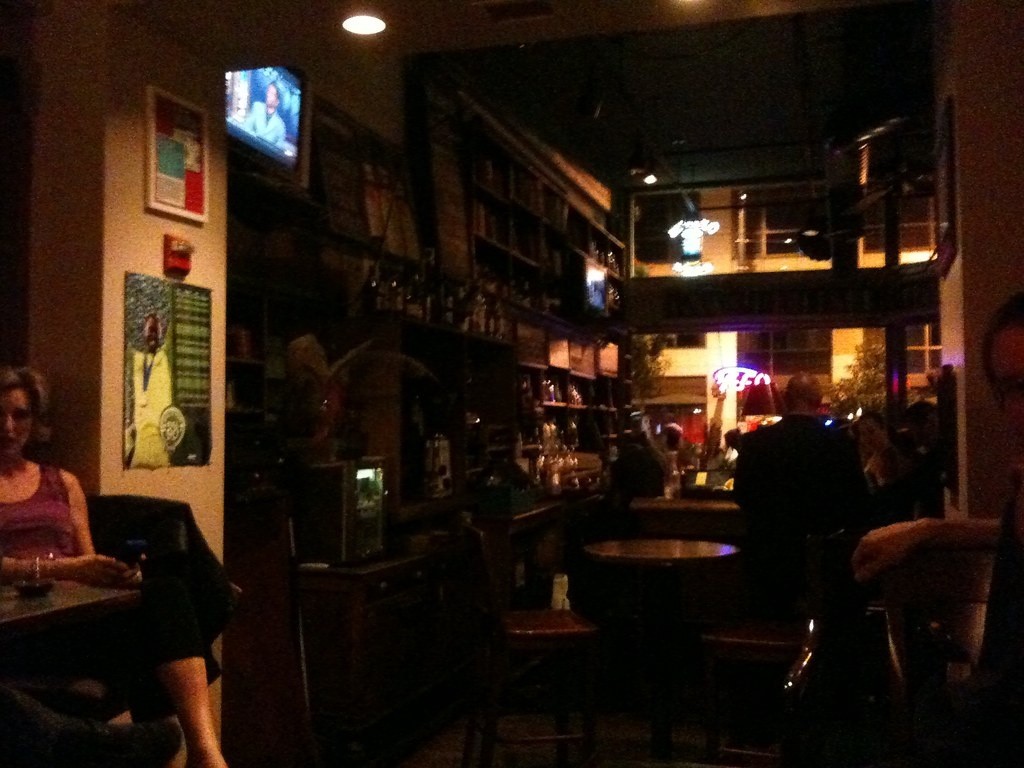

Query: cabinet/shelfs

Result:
[227,95,633,768]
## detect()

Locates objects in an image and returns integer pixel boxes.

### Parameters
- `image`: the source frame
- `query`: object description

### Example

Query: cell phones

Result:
[115,539,147,566]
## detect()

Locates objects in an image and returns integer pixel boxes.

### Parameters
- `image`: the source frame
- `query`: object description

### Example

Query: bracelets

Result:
[35,556,40,582]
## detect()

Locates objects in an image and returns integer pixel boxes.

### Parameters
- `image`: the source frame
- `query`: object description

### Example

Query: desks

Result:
[0,577,142,644]
[584,538,741,758]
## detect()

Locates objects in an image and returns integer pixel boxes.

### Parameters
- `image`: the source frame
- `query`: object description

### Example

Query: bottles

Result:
[426,283,470,331]
[370,266,425,321]
[470,288,503,340]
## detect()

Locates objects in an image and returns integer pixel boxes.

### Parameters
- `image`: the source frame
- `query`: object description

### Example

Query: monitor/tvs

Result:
[225,64,304,169]
[586,264,608,311]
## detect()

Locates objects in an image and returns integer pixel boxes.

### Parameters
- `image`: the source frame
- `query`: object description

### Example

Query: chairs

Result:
[43,494,234,721]
[702,528,864,761]
[461,522,599,768]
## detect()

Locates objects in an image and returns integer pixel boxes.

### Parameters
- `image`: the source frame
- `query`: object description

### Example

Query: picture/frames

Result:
[934,96,955,275]
[143,85,208,224]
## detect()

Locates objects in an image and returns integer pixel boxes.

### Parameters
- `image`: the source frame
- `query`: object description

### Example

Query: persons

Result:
[1,364,230,768]
[932,289,1023,768]
[247,81,287,151]
[736,371,887,724]
[823,396,945,528]
[603,419,686,538]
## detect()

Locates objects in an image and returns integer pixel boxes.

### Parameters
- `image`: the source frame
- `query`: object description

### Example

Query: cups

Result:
[14,551,52,598]
